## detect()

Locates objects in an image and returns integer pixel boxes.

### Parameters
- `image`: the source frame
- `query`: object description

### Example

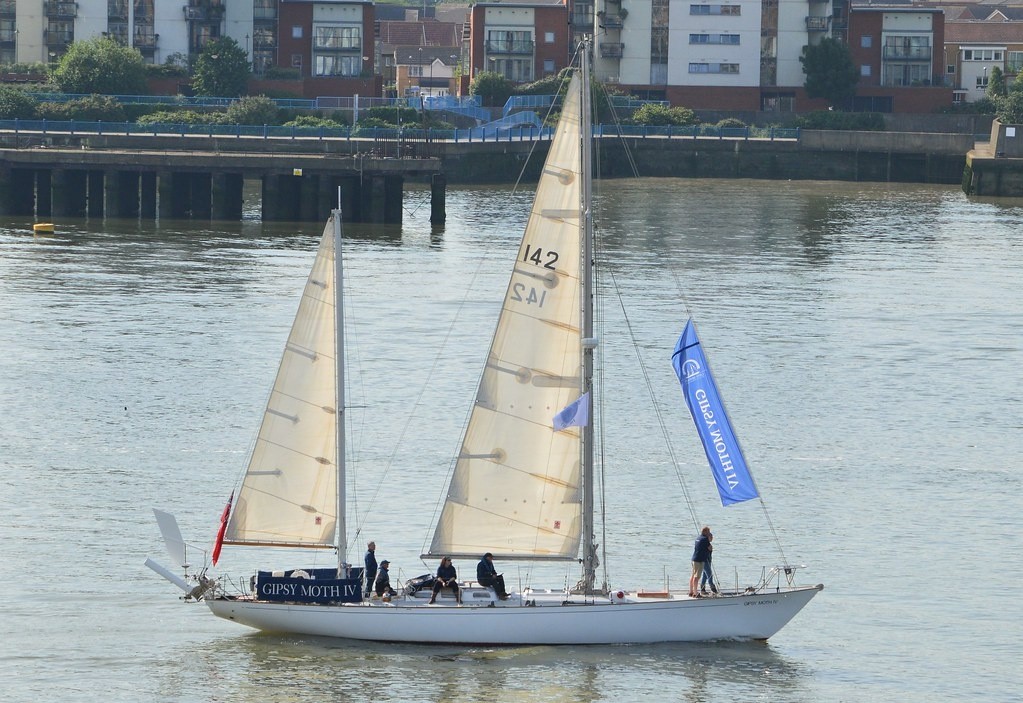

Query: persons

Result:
[476,552,511,601]
[364,541,378,598]
[376,559,397,596]
[428,556,464,604]
[688,526,721,599]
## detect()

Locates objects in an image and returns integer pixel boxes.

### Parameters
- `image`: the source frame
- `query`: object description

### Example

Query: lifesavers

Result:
[292,570,310,579]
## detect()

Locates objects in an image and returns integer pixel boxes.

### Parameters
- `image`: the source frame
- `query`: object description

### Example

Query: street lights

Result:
[490,57,497,107]
[14,28,21,73]
[396,105,404,159]
[49,49,56,95]
[418,46,424,97]
[408,54,413,97]
[450,54,458,95]
[429,56,436,109]
[419,91,432,159]
[211,53,219,98]
[361,55,370,129]
[245,33,250,61]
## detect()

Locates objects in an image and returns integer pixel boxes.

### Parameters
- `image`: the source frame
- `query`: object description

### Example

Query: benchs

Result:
[414,587,499,601]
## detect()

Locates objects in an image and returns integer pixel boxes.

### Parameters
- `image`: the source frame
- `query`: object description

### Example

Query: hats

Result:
[380,560,390,566]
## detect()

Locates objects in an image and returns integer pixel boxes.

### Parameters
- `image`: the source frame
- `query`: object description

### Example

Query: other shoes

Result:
[688,592,703,598]
[499,592,511,600]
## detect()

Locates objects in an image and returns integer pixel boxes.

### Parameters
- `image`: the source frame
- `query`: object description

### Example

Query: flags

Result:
[552,391,590,433]
[212,491,233,566]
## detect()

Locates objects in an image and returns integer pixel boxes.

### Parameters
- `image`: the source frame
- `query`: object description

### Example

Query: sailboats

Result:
[139,34,828,647]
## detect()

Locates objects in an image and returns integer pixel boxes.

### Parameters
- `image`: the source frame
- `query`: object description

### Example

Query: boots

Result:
[429,593,436,604]
[455,592,463,604]
[701,589,708,595]
[712,588,719,595]
